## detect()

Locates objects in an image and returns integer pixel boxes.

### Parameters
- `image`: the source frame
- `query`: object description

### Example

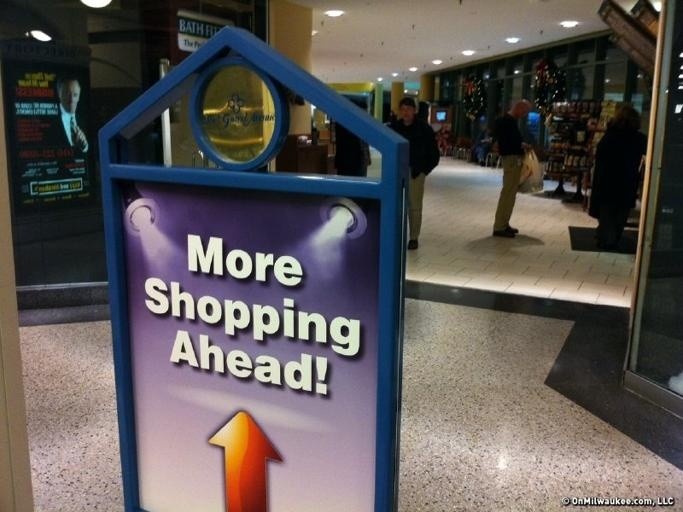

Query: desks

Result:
[542,167,590,203]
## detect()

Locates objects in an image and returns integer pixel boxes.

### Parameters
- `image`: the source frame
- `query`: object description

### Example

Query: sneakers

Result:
[406,239,418,251]
[492,225,519,239]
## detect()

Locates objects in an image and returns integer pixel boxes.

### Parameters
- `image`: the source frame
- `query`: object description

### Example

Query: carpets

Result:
[568,226,638,253]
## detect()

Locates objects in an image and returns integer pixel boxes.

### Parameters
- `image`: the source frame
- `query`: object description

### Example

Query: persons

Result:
[384,97,441,251]
[584,104,649,250]
[475,128,492,166]
[44,76,88,152]
[435,123,452,157]
[490,100,536,239]
[332,101,372,177]
